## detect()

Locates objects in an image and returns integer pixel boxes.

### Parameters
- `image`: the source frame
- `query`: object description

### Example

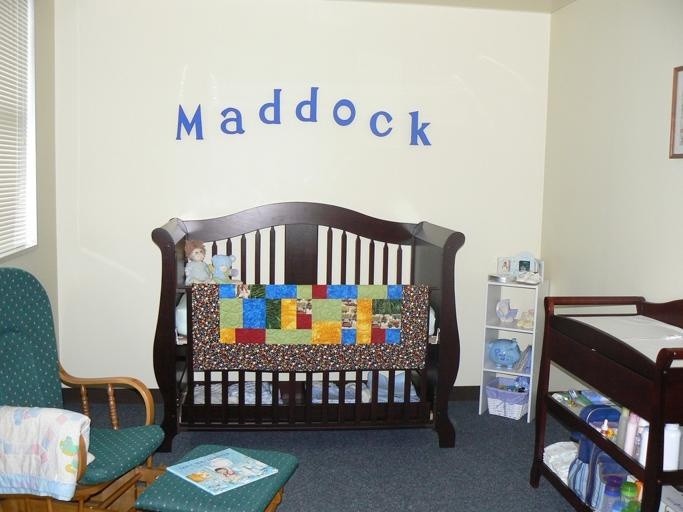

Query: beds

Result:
[148,202,466,448]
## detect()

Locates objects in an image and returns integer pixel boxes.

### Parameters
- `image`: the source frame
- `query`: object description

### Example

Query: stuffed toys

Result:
[211,254,244,285]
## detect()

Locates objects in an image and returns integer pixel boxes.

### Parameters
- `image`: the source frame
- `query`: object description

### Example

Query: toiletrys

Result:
[624,412,639,455]
[615,406,629,450]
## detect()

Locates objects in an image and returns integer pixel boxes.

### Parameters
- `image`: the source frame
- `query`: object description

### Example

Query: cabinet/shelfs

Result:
[530,296,683,512]
[478,274,542,423]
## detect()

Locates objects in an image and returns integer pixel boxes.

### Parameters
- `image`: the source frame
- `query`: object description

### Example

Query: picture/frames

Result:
[670,65,683,159]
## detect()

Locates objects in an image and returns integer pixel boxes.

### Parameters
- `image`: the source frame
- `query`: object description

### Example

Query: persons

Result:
[184,239,216,287]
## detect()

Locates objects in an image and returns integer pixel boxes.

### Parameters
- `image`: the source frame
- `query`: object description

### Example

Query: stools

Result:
[131,443,299,511]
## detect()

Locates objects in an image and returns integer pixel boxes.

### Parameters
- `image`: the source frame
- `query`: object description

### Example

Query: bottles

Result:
[602,475,641,512]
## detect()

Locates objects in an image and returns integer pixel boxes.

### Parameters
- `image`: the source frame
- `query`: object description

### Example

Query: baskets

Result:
[485,376,529,420]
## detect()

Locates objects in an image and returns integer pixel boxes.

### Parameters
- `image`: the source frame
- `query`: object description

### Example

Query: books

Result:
[165,447,279,497]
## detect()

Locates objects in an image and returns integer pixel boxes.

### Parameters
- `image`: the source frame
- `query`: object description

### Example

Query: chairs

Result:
[1,268,164,512]
